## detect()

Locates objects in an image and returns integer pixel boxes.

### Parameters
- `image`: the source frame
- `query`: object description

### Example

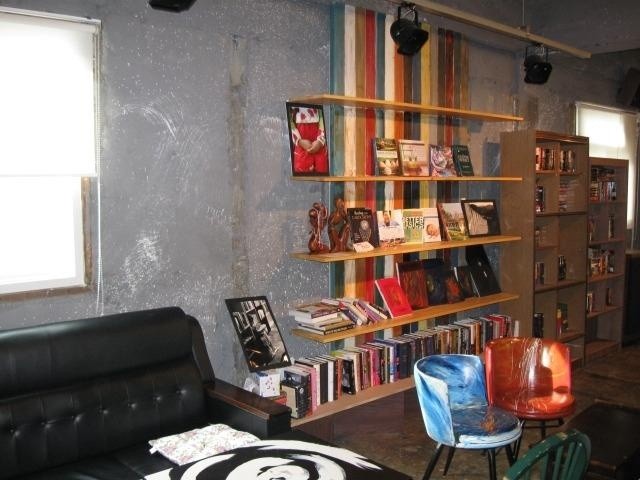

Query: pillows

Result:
[149,423,261,466]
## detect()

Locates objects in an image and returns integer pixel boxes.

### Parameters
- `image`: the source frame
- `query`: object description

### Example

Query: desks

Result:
[528,399,640,480]
[144,429,413,479]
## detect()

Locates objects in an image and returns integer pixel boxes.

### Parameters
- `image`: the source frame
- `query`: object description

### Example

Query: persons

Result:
[291,106,328,173]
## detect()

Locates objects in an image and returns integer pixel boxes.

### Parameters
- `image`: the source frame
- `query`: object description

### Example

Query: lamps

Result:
[524,42,553,85]
[390,2,429,57]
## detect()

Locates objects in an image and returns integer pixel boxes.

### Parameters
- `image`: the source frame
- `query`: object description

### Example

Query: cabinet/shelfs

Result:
[501,129,590,363]
[291,93,524,429]
[586,156,629,361]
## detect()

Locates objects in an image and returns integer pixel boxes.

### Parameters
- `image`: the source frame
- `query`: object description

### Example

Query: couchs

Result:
[0,306,291,479]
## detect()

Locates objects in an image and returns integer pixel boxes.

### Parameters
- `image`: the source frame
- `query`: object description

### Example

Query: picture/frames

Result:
[465,245,501,297]
[461,199,501,237]
[225,296,292,373]
[286,103,329,177]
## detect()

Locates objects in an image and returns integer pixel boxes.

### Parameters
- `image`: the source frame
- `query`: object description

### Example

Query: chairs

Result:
[485,337,575,438]
[414,354,522,479]
[501,430,593,480]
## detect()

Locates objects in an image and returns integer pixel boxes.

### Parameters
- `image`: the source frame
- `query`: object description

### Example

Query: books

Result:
[267,314,514,427]
[534,148,577,340]
[375,136,473,176]
[294,246,502,335]
[348,200,500,253]
[585,164,621,347]
